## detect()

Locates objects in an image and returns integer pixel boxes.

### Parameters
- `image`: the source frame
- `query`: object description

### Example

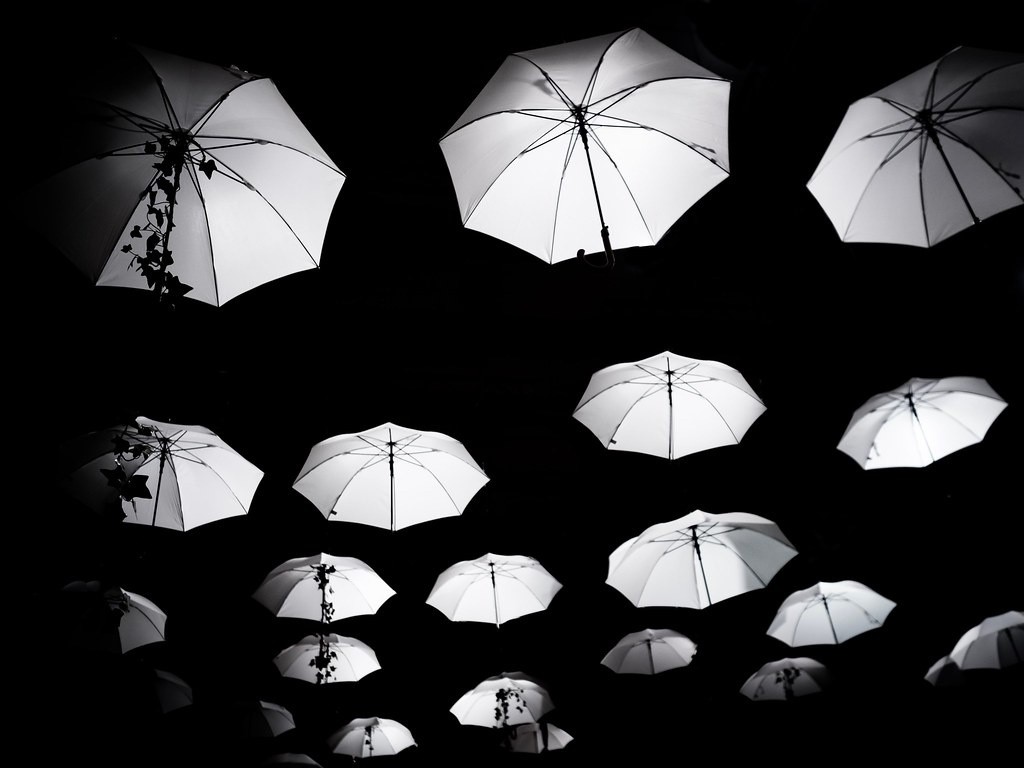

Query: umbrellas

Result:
[605,509,800,610]
[25,45,347,309]
[572,349,766,462]
[437,27,731,264]
[71,551,1024,767]
[291,422,492,531]
[63,416,264,532]
[833,376,1011,472]
[803,46,1024,246]
